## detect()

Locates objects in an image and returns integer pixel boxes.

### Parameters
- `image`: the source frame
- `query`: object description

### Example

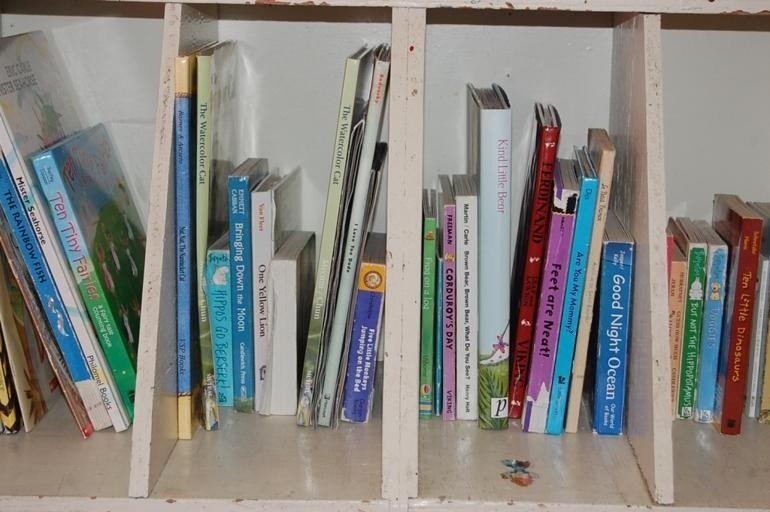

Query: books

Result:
[419,83,635,437]
[667,191,769,435]
[176,41,393,440]
[0,29,148,438]
